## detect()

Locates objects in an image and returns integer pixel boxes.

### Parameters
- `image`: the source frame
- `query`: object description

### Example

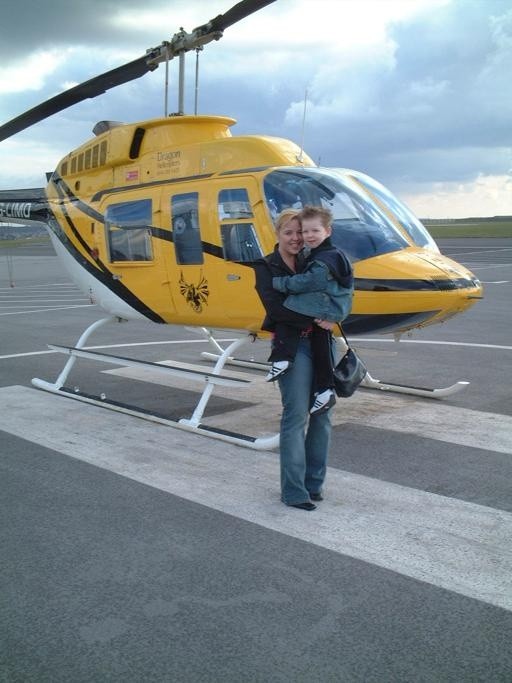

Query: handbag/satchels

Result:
[324,320,367,399]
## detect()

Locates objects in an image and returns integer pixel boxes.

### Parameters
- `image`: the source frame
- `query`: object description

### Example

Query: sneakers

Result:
[264,360,291,382]
[308,388,336,416]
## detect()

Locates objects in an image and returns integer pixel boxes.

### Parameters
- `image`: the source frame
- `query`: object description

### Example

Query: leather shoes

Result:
[294,492,323,511]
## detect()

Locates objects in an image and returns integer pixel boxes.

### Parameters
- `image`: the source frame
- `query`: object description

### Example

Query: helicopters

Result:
[0,9,487,451]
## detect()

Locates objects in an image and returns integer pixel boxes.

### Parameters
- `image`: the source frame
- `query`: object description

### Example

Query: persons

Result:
[252,206,336,510]
[265,206,354,416]
